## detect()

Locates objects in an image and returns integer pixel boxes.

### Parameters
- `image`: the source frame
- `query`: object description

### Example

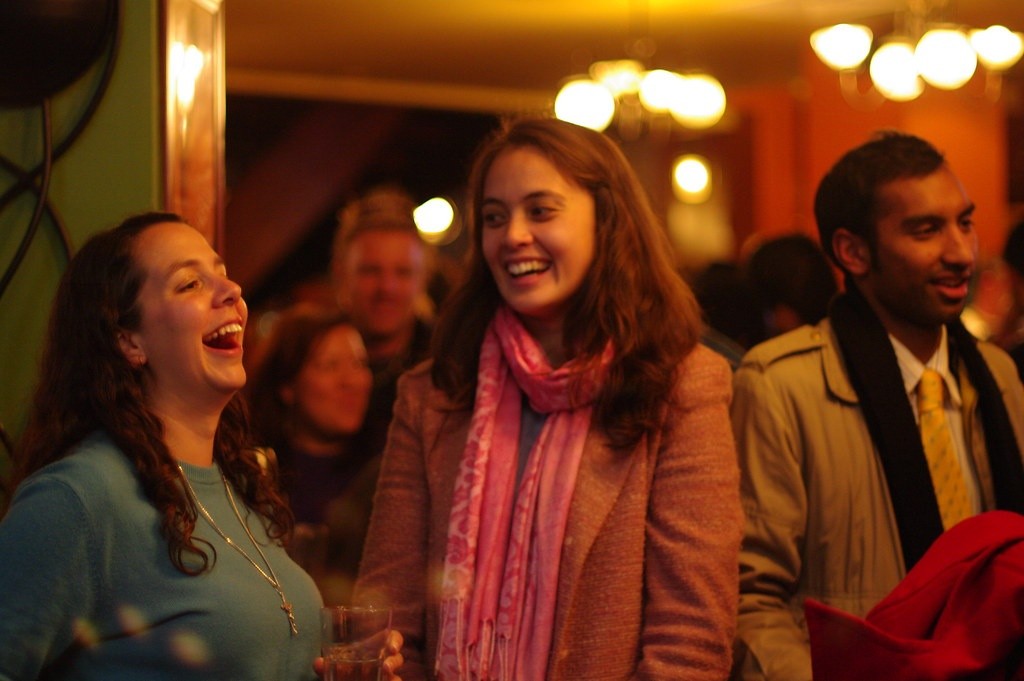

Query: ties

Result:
[915,369,976,534]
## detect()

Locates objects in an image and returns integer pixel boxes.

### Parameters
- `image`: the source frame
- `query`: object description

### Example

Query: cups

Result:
[318,605,393,681]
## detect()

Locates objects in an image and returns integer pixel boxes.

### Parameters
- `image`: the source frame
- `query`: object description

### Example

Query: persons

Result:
[733,131,1022,681]
[695,216,1023,376]
[243,178,459,546]
[0,209,406,681]
[354,115,742,681]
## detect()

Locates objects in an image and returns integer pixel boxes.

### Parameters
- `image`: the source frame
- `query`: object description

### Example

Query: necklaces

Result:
[175,464,301,636]
